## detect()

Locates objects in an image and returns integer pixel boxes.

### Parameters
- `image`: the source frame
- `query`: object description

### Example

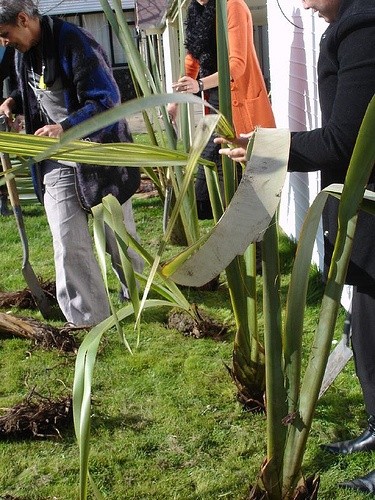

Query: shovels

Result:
[0,116,55,320]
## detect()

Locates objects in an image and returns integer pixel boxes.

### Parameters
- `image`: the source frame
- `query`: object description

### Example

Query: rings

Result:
[187,85,190,90]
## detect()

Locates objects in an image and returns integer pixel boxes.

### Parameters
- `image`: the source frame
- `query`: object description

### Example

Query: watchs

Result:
[196,78,204,91]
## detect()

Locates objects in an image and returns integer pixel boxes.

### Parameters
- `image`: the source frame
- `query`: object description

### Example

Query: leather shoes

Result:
[339,469,375,495]
[319,423,375,455]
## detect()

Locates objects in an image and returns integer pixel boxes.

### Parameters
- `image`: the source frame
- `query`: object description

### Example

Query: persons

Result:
[0,0,146,326]
[213,0,375,496]
[163,0,277,221]
